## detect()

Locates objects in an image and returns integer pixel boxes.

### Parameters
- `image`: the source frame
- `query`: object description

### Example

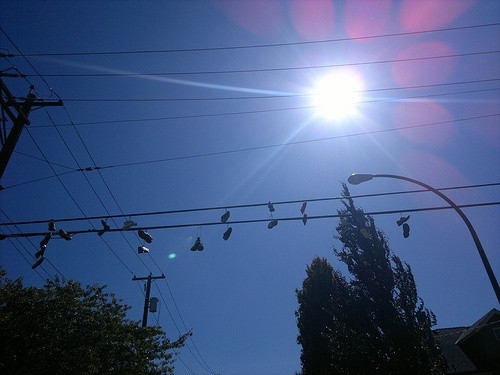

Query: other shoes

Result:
[268,220,278,229]
[123,221,137,229]
[191,237,203,251]
[300,203,306,214]
[35,245,46,258]
[396,215,410,226]
[32,257,44,269]
[221,211,230,224]
[138,230,153,243]
[98,230,105,236]
[303,214,308,224]
[223,228,232,240]
[59,229,72,240]
[268,202,275,212]
[40,234,50,247]
[49,222,55,232]
[138,245,148,253]
[101,220,110,229]
[403,223,410,238]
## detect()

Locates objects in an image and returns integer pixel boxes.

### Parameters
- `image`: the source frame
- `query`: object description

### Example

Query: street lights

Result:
[348,173,500,306]
